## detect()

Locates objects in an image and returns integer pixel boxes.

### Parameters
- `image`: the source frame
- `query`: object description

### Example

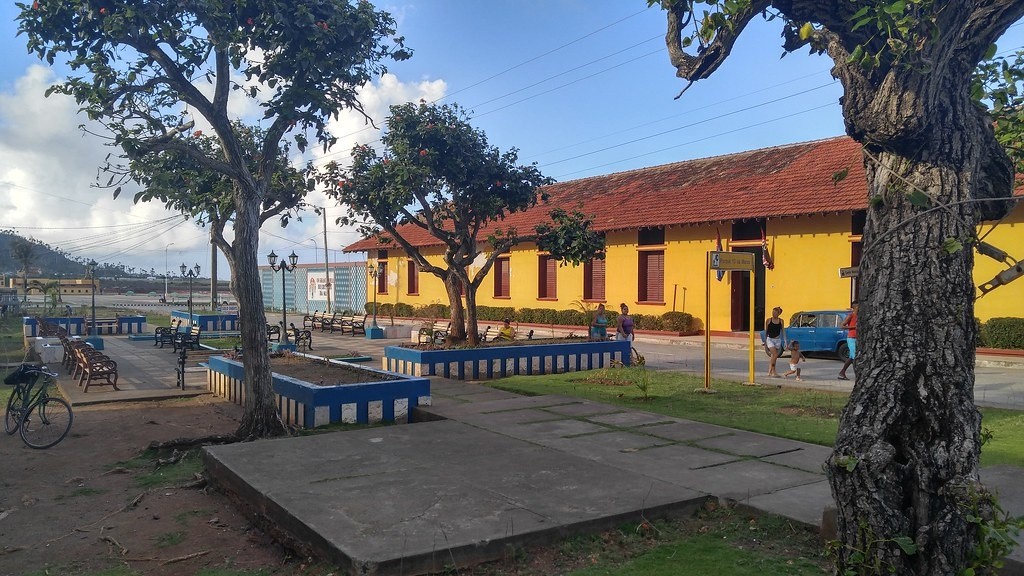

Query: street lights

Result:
[310,238,318,264]
[114,275,122,295]
[266,249,300,353]
[180,262,201,335]
[54,272,66,303]
[85,258,104,349]
[365,264,385,340]
[315,206,332,314]
[163,242,175,294]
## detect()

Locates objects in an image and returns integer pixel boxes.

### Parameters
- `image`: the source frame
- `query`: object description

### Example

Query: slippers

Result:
[838,376,849,380]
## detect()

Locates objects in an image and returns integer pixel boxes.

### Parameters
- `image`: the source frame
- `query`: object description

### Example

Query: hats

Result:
[504,318,510,325]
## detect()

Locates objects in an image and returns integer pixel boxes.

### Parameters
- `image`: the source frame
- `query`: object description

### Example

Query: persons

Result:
[766,306,787,377]
[784,338,806,381]
[616,303,634,357]
[493,317,515,341]
[837,300,860,380]
[590,303,608,338]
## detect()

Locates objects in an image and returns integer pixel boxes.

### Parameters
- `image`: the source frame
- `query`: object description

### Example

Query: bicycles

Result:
[4,361,74,450]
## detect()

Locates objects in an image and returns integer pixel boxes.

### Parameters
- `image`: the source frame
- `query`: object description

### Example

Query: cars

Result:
[760,309,854,365]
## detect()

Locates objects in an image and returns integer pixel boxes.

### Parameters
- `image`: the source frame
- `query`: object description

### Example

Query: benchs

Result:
[35,316,66,338]
[266,321,313,351]
[304,310,369,337]
[154,318,203,353]
[56,331,119,393]
[419,320,533,345]
[83,319,119,335]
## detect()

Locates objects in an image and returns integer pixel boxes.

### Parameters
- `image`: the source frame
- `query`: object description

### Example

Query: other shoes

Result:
[796,377,803,381]
[767,374,780,377]
[783,373,787,379]
